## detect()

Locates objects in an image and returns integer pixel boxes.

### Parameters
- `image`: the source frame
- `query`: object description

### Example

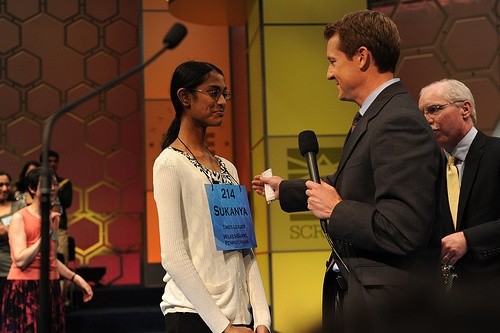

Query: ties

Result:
[351,111,361,133]
[445,156,460,230]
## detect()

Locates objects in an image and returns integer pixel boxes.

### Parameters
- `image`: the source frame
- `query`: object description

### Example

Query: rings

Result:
[444,255,450,260]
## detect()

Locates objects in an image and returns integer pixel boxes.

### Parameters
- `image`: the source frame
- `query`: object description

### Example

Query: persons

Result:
[251,11,439,333]
[417,79,500,333]
[152,60,270,333]
[0,149,93,333]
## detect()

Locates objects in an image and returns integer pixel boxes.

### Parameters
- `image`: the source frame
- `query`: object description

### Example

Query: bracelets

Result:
[71,273,77,282]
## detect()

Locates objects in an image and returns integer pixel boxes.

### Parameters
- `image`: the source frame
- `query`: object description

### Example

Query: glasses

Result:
[189,89,232,100]
[424,100,465,115]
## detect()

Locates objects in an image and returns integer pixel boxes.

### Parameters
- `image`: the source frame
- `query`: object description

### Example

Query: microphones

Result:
[298,130,328,233]
[50,196,61,234]
[39,22,189,194]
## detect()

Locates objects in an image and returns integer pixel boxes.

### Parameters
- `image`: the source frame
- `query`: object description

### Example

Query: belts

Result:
[336,276,349,290]
